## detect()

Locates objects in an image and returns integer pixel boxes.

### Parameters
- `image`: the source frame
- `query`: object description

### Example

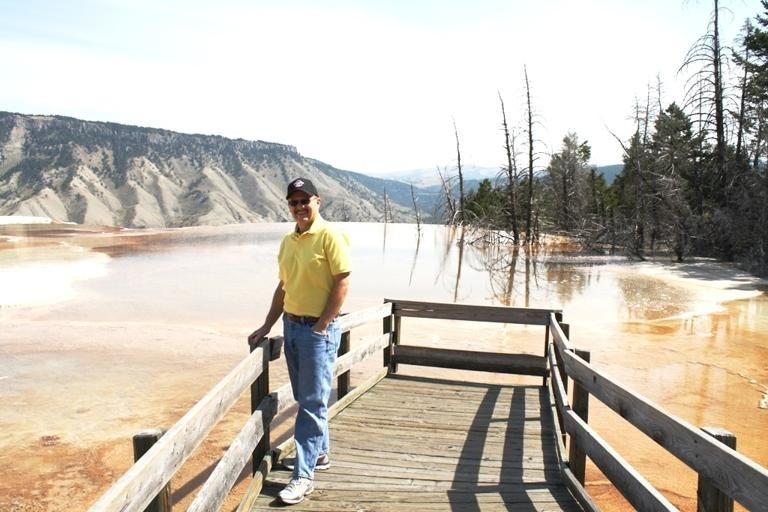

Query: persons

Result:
[247,177,351,505]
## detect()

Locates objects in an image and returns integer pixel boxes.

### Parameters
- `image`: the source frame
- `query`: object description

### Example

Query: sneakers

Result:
[280,454,331,470]
[275,476,316,505]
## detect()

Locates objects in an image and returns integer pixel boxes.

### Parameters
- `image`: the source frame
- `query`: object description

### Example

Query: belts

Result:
[284,312,318,325]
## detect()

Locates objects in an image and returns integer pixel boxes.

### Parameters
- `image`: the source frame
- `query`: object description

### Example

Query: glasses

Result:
[287,198,312,206]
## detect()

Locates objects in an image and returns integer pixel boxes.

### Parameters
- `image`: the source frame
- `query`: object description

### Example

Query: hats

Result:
[286,177,319,200]
